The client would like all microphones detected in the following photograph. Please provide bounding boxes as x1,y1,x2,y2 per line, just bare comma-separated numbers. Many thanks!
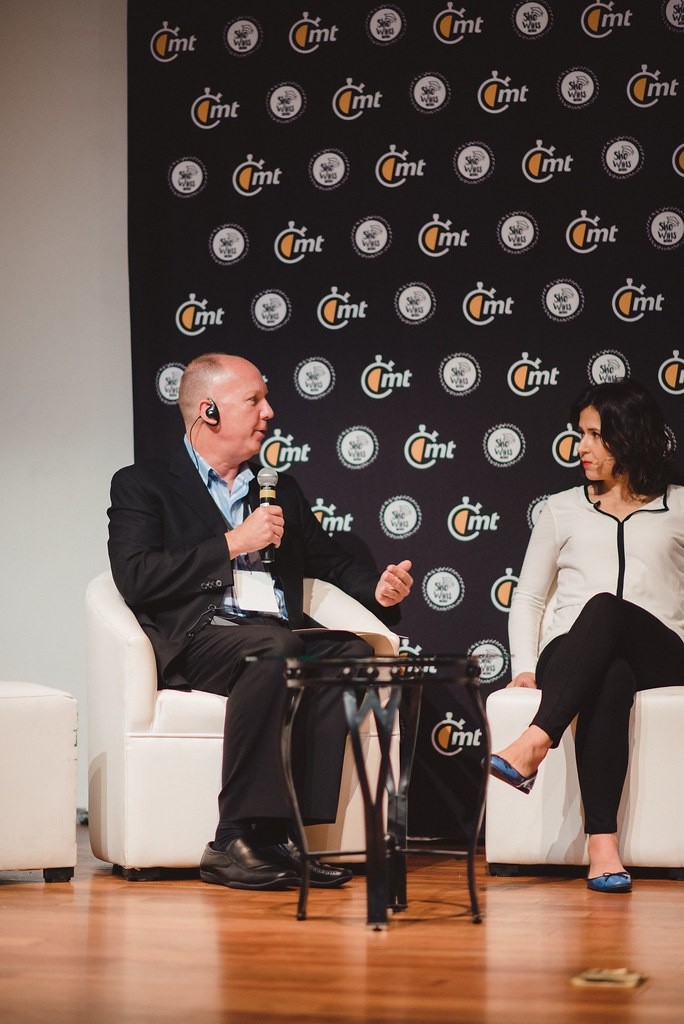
593,458,614,466
256,467,279,563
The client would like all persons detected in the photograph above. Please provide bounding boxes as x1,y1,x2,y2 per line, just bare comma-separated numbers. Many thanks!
107,354,413,886
481,381,684,892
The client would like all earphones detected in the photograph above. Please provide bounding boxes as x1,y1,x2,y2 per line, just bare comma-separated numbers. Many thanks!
206,400,219,422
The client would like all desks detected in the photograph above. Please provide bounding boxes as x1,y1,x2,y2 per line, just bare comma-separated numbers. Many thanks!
284,654,495,932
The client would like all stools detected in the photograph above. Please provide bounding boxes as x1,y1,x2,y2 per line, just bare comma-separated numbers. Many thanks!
485,685,684,880
0,681,79,883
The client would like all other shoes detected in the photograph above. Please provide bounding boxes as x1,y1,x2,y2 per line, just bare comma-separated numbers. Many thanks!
588,863,632,891
481,754,538,793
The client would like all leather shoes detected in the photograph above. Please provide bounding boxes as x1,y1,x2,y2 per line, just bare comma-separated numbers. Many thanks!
200,835,300,890
249,837,350,887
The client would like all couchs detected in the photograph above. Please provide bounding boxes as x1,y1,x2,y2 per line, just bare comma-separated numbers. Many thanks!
89,566,400,882
